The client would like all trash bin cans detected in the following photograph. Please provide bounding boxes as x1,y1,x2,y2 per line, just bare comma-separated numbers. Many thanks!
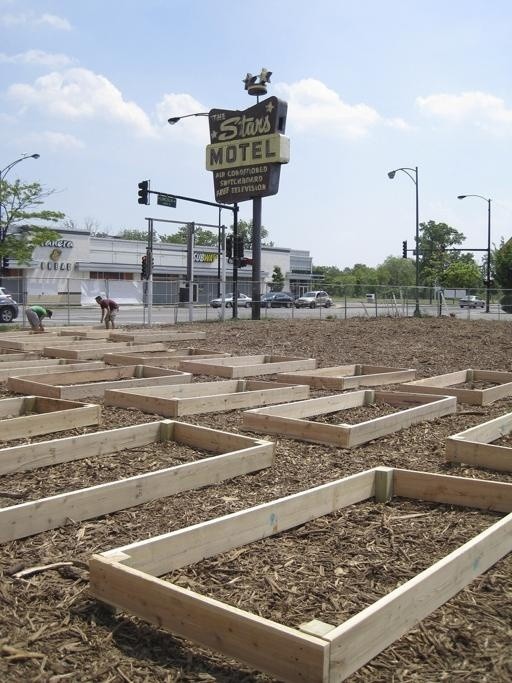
366,294,375,304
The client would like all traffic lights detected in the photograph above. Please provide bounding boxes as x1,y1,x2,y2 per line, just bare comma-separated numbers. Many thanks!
137,178,151,205
402,240,407,258
141,257,147,273
237,236,245,256
151,257,155,268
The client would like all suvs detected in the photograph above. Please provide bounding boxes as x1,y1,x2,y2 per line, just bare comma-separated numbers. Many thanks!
295,289,332,308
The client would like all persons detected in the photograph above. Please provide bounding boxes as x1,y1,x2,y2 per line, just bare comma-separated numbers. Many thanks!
94,296,119,329
25,305,53,330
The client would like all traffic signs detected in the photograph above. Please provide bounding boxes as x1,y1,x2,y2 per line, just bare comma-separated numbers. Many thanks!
156,194,178,208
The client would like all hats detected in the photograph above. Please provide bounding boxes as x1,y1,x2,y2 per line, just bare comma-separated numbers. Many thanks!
47,310,53,319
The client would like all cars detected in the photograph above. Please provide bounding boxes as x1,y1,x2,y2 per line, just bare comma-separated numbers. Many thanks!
458,294,485,308
210,291,253,307
261,291,294,307
0,286,18,323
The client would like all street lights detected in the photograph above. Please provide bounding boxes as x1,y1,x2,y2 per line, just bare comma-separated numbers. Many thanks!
0,151,40,190
387,164,421,317
168,109,240,320
457,193,491,312
